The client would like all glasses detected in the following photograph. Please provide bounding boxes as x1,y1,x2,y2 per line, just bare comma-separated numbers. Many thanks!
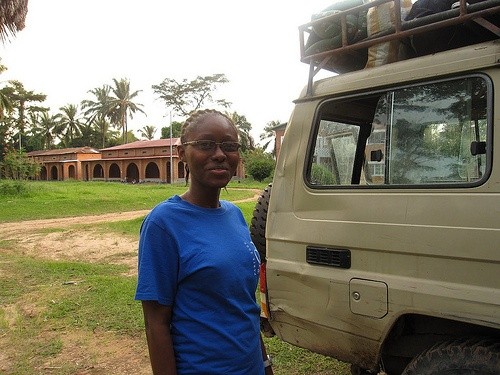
182,140,242,152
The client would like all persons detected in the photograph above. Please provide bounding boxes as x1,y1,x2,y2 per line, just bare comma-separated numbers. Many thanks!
134,108,275,375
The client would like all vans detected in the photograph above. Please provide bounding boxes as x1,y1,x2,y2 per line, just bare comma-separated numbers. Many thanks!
246,0,499,375
